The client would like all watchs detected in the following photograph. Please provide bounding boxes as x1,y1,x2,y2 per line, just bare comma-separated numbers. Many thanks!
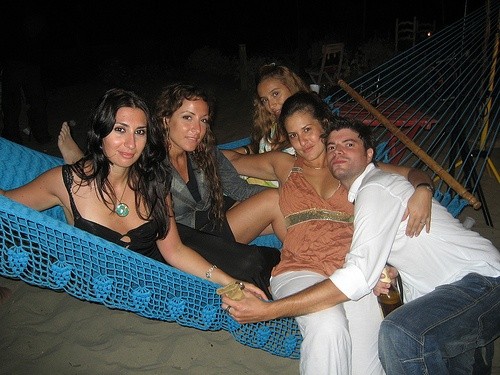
415,182,434,198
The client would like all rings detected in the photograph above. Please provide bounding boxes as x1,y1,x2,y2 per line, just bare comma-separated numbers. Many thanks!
225,305,231,312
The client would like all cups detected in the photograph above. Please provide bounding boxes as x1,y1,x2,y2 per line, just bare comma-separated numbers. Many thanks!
310,84,320,95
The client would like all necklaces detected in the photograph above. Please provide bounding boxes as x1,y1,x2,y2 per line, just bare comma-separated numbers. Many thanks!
303,162,328,169
108,184,129,218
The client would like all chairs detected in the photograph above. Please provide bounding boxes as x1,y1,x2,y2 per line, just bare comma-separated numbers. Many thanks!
395,17,416,51
308,43,344,84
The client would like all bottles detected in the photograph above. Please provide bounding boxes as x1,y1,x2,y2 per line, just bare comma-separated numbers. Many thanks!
377,265,401,318
371,73,380,106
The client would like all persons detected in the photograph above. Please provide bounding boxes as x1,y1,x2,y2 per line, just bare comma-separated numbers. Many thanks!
0,88,281,320
151,82,286,243
222,92,435,375
59,63,309,188
222,116,500,375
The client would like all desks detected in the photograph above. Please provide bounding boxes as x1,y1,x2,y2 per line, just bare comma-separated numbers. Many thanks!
334,97,437,166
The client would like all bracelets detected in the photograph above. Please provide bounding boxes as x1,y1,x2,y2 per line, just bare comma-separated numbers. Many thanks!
235,280,245,290
206,264,217,279
